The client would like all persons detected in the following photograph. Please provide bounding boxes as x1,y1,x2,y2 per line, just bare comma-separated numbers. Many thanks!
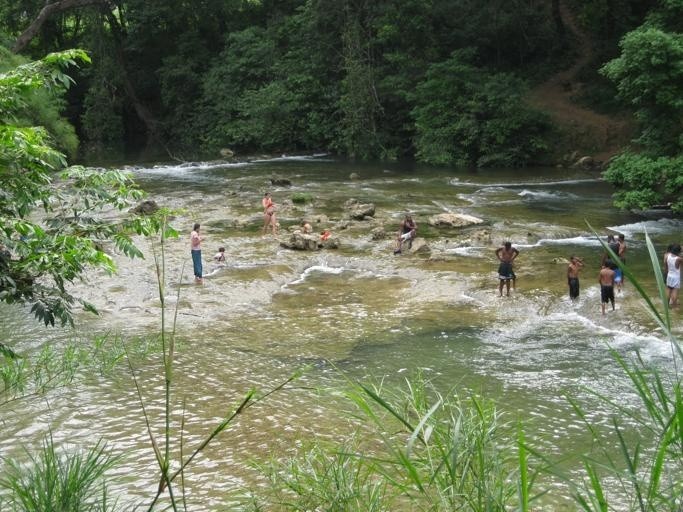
394,215,417,253
496,234,683,315
262,193,276,236
214,248,226,260
191,224,204,280
301,222,313,233
